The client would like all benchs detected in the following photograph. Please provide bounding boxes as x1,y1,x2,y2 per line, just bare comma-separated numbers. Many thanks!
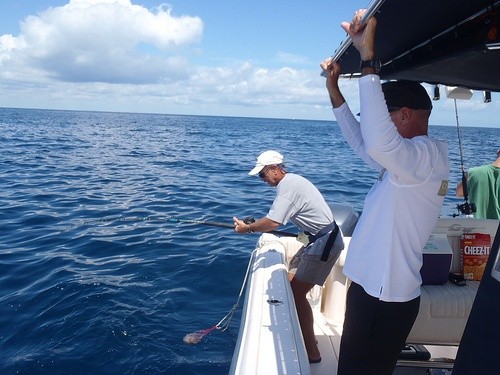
405,236,481,345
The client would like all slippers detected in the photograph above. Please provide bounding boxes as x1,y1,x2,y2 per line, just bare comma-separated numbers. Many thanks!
308,357,321,363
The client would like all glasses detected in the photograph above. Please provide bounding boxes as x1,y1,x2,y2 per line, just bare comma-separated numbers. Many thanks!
259,168,269,178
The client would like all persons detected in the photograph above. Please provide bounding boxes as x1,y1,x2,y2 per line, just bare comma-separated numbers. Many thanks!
233,150,344,364
456,150,500,220
320,8,449,375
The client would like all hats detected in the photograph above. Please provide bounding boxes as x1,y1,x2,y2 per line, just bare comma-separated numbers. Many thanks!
357,80,432,116
248,151,283,175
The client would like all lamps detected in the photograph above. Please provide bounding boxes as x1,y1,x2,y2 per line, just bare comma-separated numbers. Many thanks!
433,84,440,100
482,90,491,103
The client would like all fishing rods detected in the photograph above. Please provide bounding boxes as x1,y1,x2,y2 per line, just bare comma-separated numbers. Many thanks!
454,98,476,215
71,215,313,246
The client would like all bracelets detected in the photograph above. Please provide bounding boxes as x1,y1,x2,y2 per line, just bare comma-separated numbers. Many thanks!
248,225,254,234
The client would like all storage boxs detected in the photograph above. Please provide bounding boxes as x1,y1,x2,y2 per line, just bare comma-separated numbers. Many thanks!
420,233,453,285
460,233,491,280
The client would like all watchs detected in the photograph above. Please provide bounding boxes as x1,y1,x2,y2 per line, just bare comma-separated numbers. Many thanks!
360,56,381,72
